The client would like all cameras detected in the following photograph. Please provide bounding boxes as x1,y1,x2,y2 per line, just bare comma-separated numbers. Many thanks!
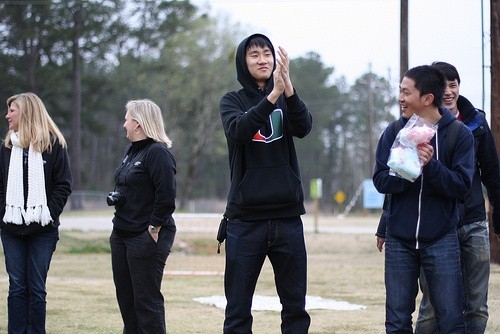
107,191,124,206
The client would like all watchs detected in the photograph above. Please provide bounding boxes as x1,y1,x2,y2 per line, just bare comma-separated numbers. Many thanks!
150,225,159,233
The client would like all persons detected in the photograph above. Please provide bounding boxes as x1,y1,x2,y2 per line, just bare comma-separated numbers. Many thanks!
217,33,313,334
373,62,500,334
0,93,73,334
107,99,177,334
